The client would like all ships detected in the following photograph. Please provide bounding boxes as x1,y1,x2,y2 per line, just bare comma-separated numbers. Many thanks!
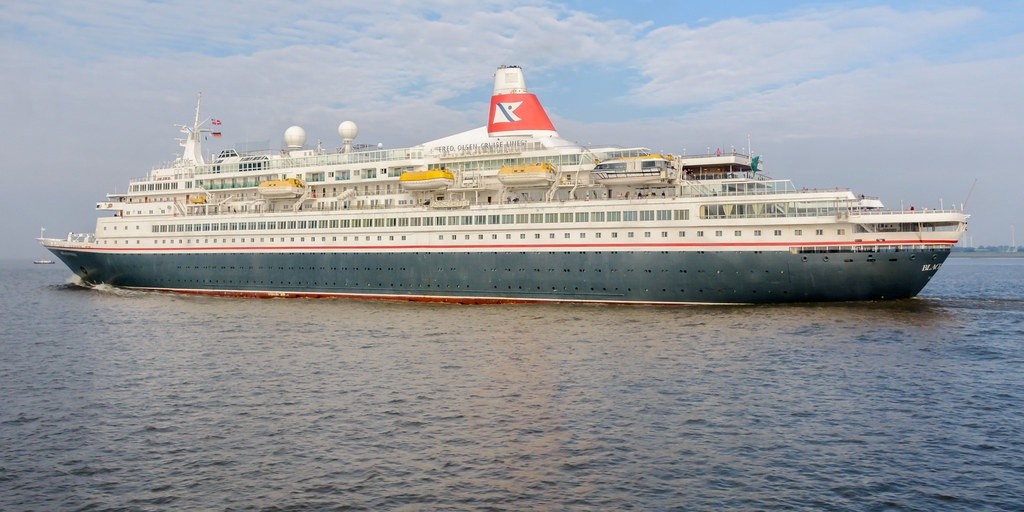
36,65,975,304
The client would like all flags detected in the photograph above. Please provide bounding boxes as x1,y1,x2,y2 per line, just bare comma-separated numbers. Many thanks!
211,119,222,138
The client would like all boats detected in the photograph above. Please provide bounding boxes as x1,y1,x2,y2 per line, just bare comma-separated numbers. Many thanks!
34,259,54,264
398,166,453,193
256,178,307,199
498,163,557,187
591,152,672,185
190,195,207,204
